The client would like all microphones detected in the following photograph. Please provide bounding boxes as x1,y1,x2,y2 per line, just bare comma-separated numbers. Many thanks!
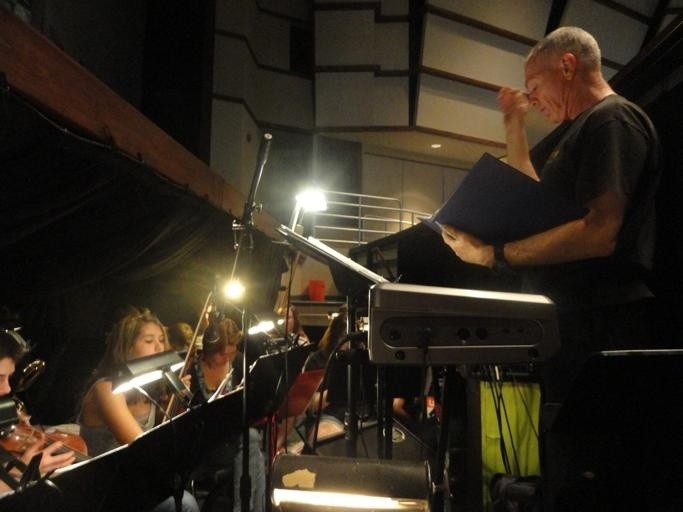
250,130,272,202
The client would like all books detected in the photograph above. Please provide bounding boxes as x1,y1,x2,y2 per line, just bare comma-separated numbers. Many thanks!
416,153,587,249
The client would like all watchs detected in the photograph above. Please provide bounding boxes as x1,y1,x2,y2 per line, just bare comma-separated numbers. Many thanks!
488,241,512,277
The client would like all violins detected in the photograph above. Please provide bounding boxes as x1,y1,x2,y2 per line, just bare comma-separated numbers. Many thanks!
0,419,95,467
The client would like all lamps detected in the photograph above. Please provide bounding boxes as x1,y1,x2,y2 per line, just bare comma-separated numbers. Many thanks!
246,312,286,338
110,349,201,420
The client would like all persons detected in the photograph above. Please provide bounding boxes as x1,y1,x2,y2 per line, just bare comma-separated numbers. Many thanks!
1,330,76,493
431,24,666,512
77,297,445,511
